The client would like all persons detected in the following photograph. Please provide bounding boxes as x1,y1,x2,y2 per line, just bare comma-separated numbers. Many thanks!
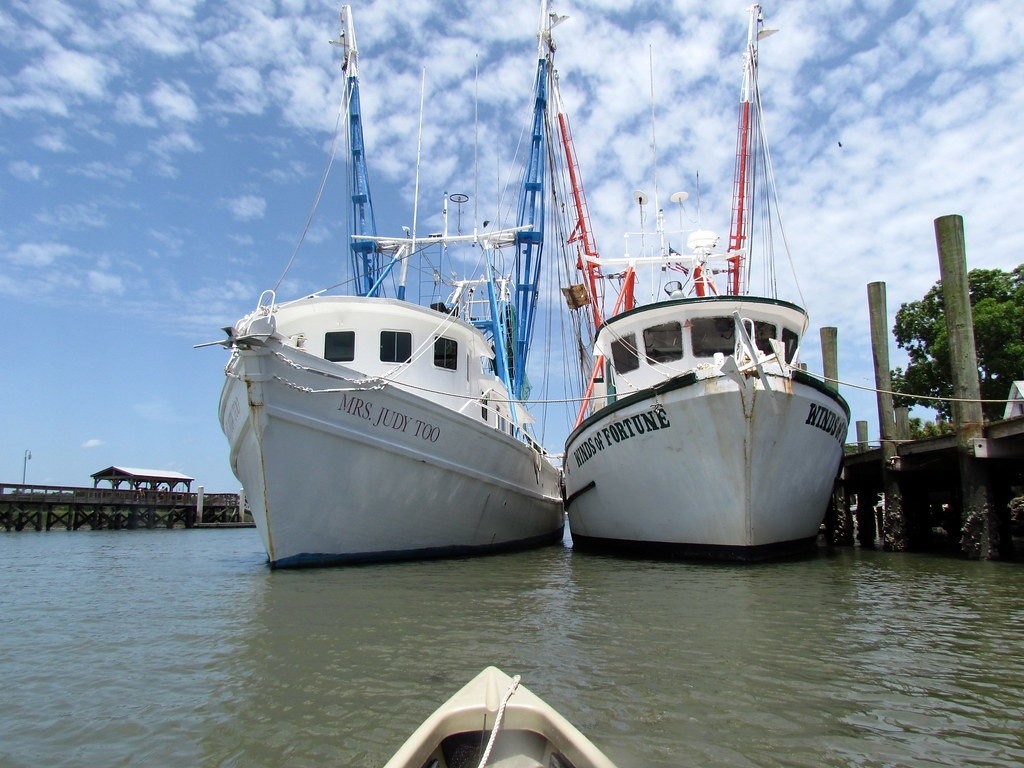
135,485,167,502
935,410,942,425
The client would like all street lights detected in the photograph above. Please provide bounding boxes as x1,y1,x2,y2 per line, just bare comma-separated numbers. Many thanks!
23,449,32,494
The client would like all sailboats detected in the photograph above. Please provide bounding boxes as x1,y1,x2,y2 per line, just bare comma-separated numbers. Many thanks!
553,1,854,567
189,0,568,572
